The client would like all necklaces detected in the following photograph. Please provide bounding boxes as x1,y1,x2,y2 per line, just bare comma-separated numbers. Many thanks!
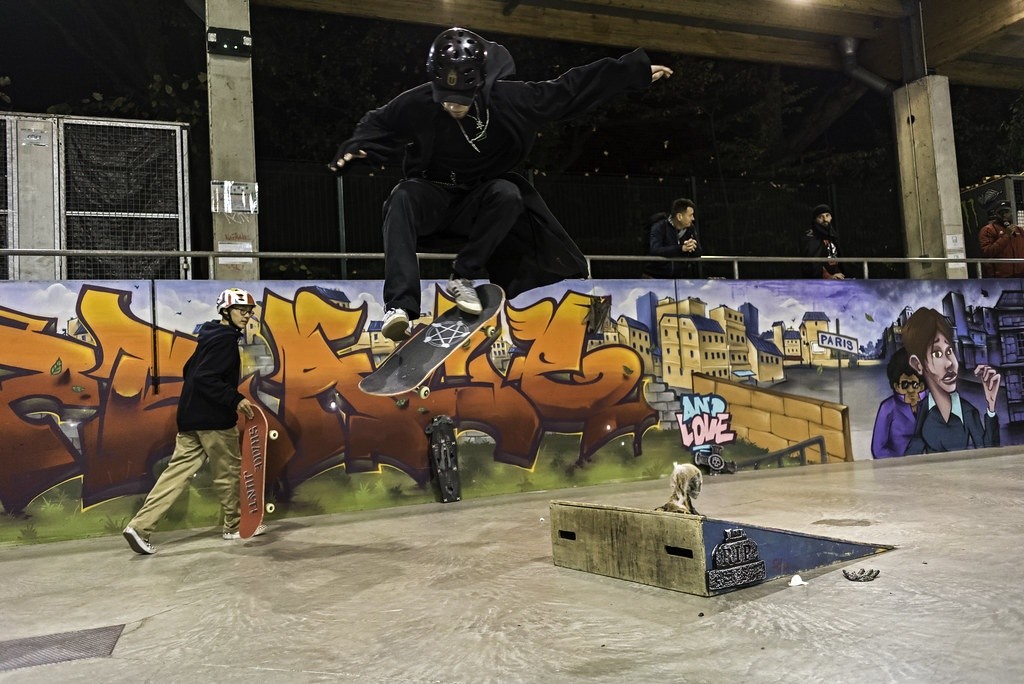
457,100,490,153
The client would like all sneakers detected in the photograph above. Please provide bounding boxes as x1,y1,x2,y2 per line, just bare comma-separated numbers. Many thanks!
381,308,411,342
446,278,482,315
122,527,157,554
222,524,268,539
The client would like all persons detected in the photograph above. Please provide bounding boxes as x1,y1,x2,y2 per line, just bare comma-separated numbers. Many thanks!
978,201,1024,278
638,198,702,279
325,28,671,341
124,288,267,555
799,205,862,280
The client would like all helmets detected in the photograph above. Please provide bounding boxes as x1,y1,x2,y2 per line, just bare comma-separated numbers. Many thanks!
216,288,255,314
427,27,486,90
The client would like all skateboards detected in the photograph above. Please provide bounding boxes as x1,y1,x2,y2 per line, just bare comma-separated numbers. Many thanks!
358,283,506,399
423,413,462,504
238,404,278,541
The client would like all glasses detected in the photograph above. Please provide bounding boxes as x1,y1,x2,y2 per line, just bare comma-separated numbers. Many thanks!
230,306,255,318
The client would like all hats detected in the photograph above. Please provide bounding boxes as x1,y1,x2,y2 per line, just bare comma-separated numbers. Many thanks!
813,205,831,220
994,200,1011,213
432,89,476,106
